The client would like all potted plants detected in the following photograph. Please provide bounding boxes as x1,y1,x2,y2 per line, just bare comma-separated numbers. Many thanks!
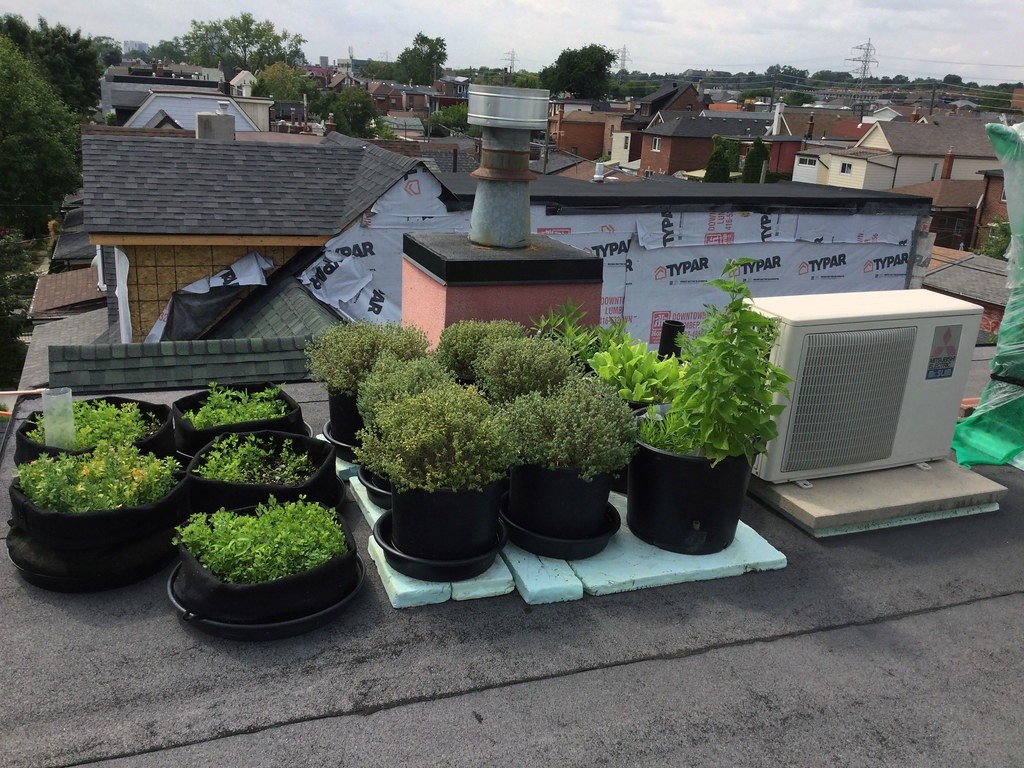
5,258,797,641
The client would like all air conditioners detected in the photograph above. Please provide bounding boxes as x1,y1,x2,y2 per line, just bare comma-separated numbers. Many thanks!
730,288,984,489
